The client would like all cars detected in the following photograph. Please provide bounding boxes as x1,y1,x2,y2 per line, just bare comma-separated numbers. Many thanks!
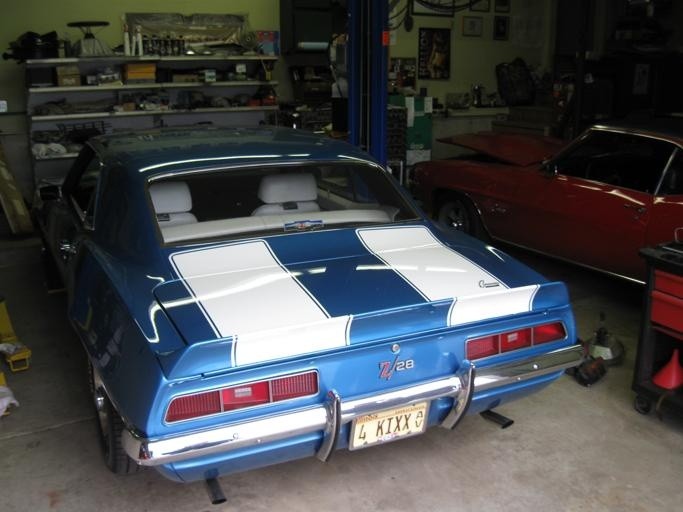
410,121,683,288
36,124,584,485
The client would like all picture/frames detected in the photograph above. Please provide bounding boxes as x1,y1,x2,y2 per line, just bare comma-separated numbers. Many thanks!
492,15,510,41
462,15,483,37
417,27,451,81
411,0,455,18
469,0,510,13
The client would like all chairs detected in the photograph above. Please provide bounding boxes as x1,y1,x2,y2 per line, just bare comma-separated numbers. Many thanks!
148,182,197,229
250,174,321,217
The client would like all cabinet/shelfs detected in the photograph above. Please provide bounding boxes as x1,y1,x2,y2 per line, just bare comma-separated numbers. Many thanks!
24,55,282,207
631,239,682,413
